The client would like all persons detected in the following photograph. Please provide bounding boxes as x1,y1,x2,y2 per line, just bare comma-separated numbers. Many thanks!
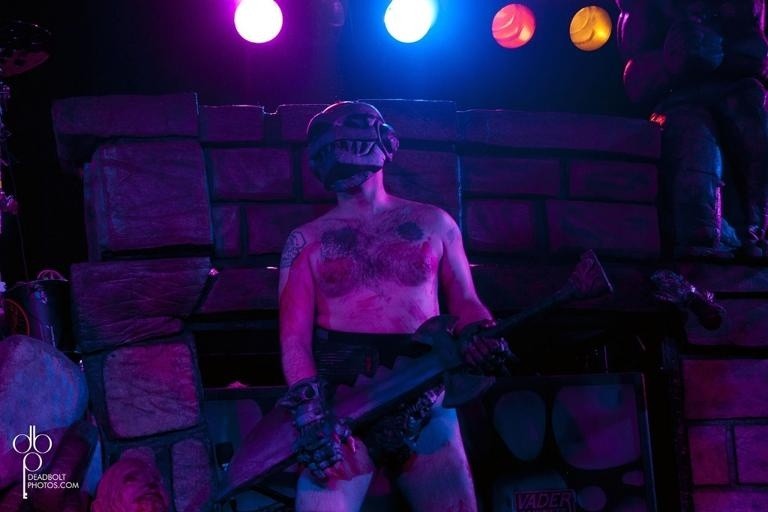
278,103,502,511
91,455,170,512
